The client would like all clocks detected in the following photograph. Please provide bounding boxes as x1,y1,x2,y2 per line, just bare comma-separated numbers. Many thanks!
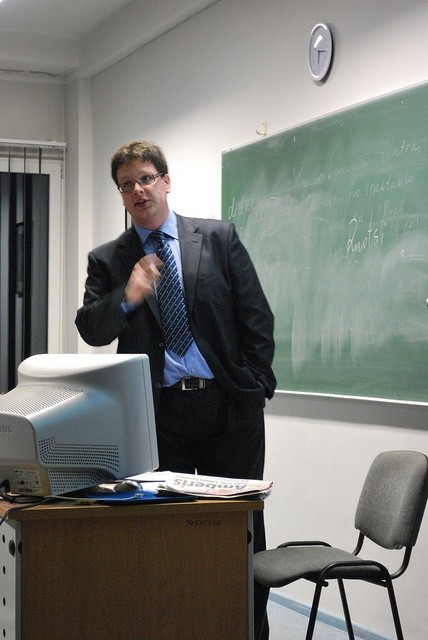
307,22,332,82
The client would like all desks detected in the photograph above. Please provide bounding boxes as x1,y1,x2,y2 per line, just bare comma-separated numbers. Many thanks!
0,496,265,639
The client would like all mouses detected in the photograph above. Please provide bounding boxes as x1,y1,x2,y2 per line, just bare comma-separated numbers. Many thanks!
114,478,143,494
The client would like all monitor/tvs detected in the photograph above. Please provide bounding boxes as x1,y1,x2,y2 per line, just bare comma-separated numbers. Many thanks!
0,353,159,497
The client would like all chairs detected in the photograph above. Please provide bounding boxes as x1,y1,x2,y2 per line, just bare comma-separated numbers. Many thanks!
252,450,428,640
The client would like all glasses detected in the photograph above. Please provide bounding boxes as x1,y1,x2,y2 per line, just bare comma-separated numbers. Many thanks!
118,171,165,194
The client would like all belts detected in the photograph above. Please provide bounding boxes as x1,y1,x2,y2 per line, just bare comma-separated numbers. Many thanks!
177,376,216,391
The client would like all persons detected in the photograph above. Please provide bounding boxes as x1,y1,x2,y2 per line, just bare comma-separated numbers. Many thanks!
75,142,277,635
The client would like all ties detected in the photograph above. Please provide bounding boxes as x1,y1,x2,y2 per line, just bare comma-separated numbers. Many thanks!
150,231,193,358
